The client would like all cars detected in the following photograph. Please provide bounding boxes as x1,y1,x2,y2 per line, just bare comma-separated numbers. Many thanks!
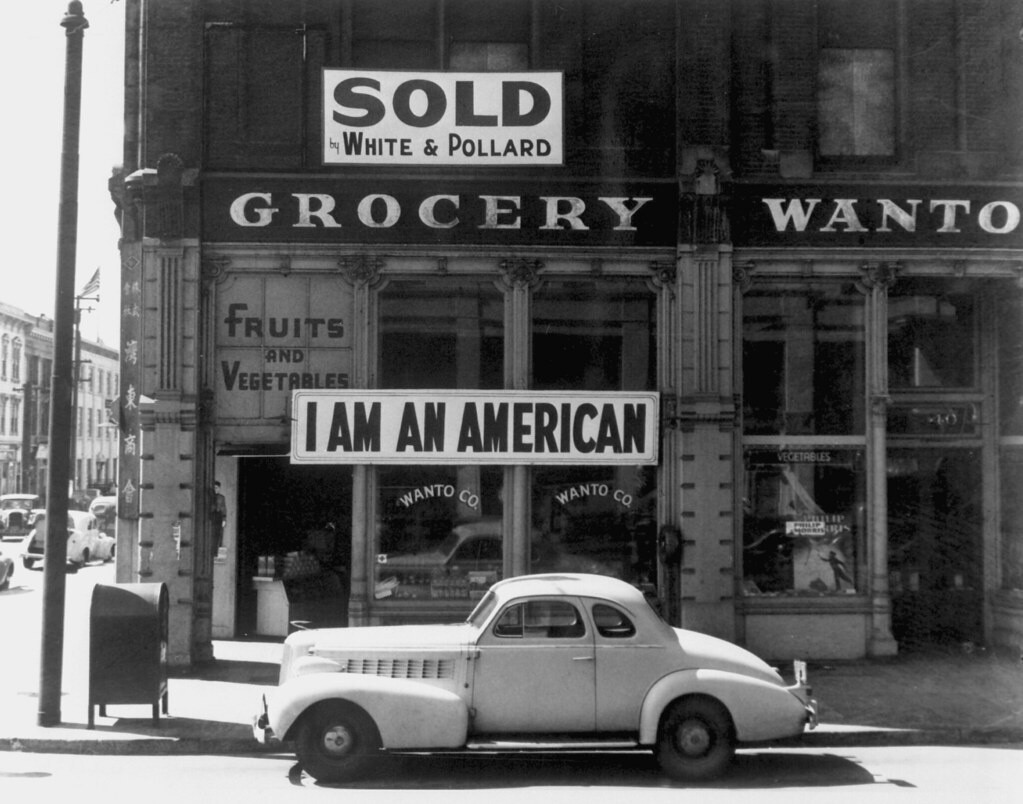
0,492,46,540
19,509,113,569
378,518,625,583
88,496,121,537
249,570,820,782
1,552,15,591
